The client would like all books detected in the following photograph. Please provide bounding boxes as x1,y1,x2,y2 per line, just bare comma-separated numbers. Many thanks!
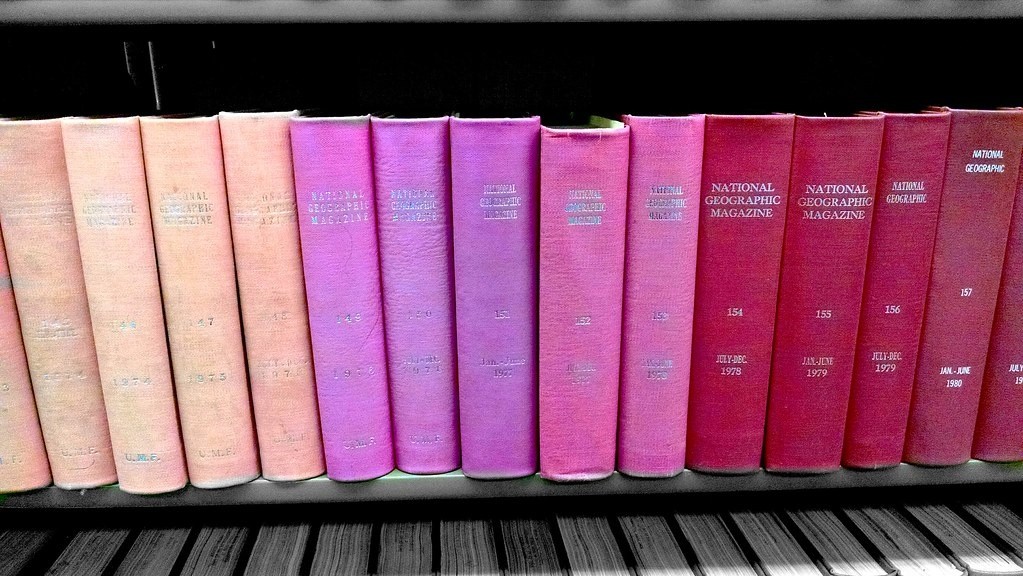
61,115,187,494
1,503,1023,576
137,103,1023,494
0,115,116,490
0,235,52,493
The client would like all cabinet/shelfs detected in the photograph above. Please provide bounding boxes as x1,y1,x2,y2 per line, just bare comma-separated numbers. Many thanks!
0,0,1023,510
